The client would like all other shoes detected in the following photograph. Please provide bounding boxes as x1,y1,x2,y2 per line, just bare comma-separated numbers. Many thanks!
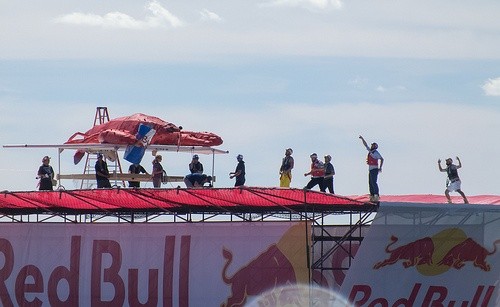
448,201,452,203
465,200,468,204
370,194,380,201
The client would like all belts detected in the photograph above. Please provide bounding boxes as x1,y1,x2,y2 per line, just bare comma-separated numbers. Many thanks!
452,179,459,183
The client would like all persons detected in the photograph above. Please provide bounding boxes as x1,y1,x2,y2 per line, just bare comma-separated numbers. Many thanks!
189,154,203,186
229,154,246,187
324,154,335,194
185,173,212,188
127,163,150,187
438,156,469,204
279,148,294,188
38,156,54,190
359,136,384,199
95,153,112,188
302,152,326,192
152,155,166,188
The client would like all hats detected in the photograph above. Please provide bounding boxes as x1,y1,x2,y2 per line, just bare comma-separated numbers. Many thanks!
446,158,453,162
310,153,317,158
193,154,198,159
207,176,212,183
237,154,243,159
96,154,102,159
42,156,51,161
324,155,332,160
286,148,293,154
371,143,378,149
